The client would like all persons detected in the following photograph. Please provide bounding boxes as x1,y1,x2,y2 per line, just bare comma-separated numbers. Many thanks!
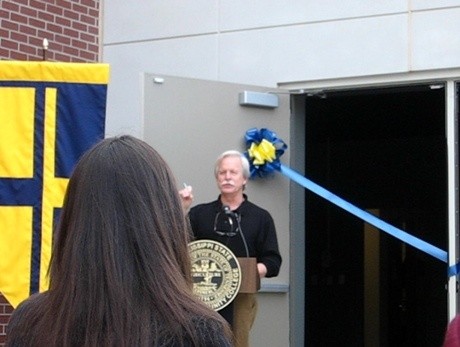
4,133,234,347
176,148,283,347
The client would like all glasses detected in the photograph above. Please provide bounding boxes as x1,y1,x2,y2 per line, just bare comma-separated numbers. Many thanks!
214,211,241,237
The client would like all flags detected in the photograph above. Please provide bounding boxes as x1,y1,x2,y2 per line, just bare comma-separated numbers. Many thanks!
0,61,111,311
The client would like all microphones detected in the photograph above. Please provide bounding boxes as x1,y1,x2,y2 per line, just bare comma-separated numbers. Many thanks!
222,205,234,230
